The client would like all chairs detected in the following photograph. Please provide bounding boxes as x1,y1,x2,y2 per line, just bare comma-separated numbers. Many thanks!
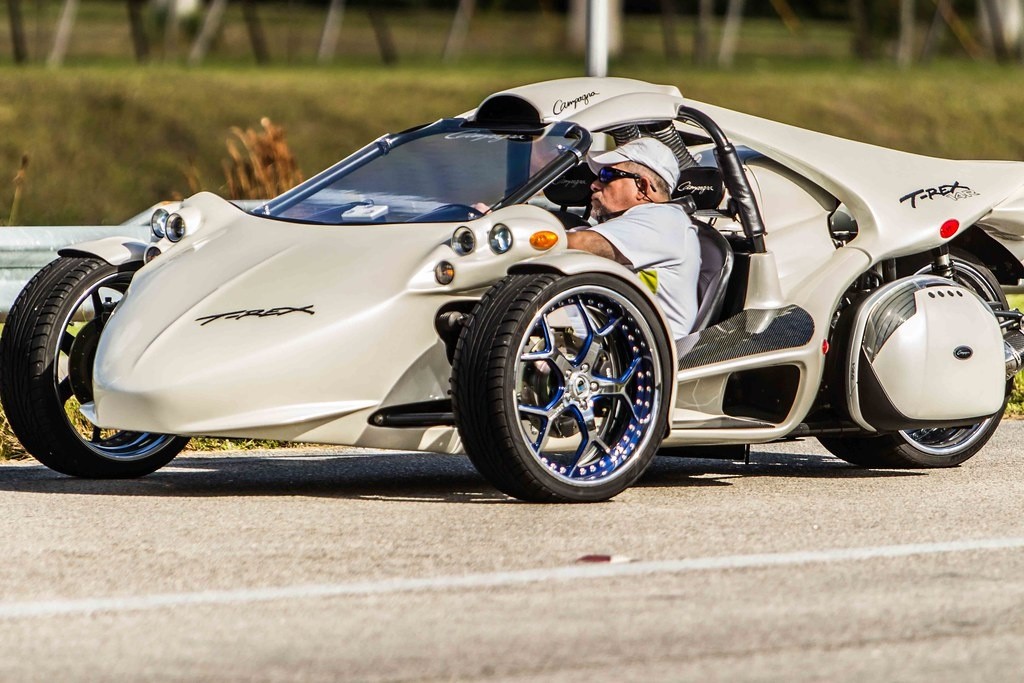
543,162,599,230
671,166,734,334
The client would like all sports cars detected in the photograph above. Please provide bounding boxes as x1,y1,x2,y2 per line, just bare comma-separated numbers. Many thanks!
0,74,1024,502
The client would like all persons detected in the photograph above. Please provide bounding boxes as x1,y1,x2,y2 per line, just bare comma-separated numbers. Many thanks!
469,136,701,340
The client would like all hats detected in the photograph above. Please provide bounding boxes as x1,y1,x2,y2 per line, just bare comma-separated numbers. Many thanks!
587,138,681,195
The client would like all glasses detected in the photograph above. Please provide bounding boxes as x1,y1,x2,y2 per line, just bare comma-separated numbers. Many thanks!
598,167,656,192
